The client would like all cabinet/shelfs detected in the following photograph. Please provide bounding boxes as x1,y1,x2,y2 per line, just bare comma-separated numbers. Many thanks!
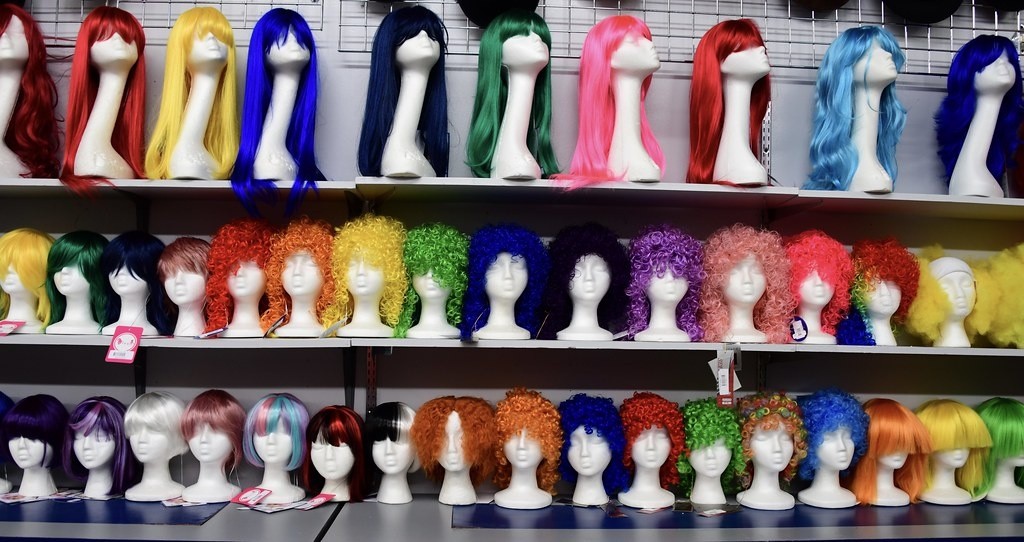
0,177,1024,542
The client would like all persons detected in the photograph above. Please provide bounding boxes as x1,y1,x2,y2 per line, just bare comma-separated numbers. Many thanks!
0,6,1024,508
